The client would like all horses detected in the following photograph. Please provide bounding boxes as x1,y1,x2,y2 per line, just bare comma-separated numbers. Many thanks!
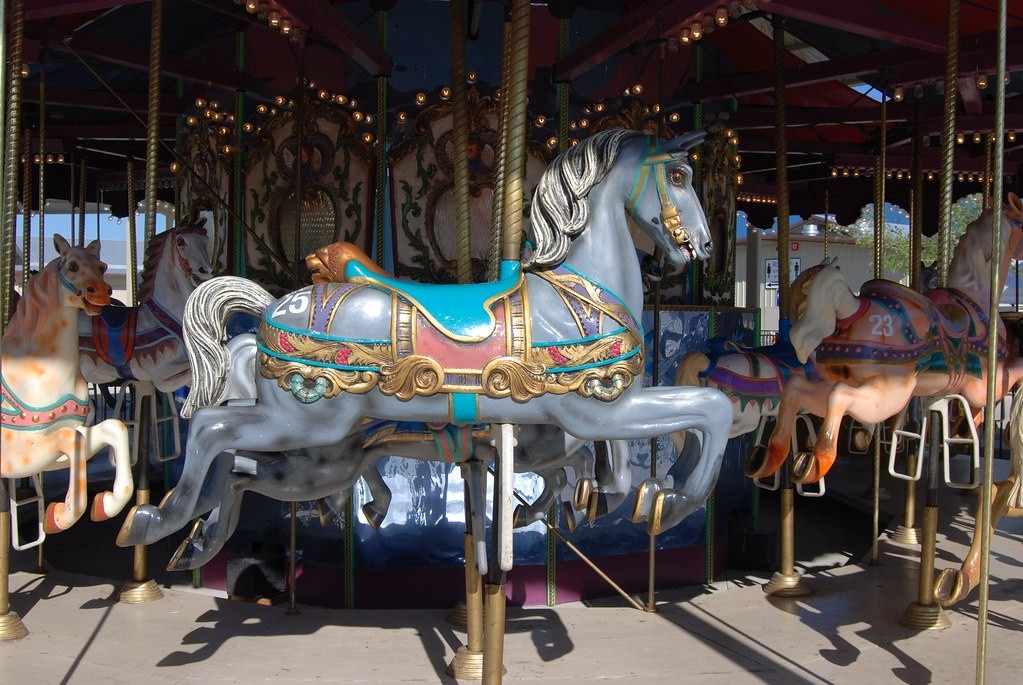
667,191,1023,484
115,128,734,572
0,214,214,534
